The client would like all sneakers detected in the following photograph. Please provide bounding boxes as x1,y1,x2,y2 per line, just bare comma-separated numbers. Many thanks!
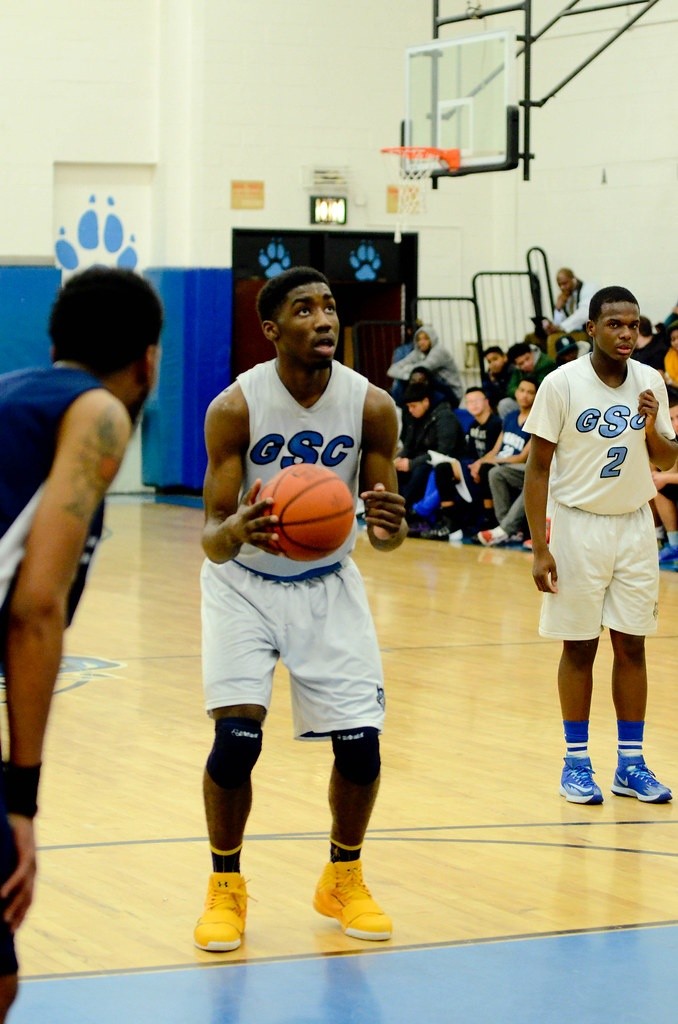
312,859,392,940
610,761,672,802
559,757,601,804
478,526,508,546
524,519,550,549
193,872,257,951
505,532,524,542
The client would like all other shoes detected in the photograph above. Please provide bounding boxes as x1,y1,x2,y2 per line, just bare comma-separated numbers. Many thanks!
658,542,678,563
449,529,463,540
408,524,431,538
421,527,450,540
471,534,480,543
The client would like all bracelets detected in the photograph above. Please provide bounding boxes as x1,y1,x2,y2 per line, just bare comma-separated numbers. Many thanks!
2,760,42,819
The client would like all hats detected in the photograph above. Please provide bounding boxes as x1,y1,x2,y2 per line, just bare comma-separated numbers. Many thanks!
557,335,578,356
396,383,431,405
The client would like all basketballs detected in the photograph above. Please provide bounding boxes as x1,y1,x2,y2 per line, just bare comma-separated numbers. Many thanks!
259,463,358,564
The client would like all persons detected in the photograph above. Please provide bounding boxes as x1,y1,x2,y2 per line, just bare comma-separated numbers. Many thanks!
522,287,678,802
0,270,161,1024
193,266,408,952
343,267,678,571
